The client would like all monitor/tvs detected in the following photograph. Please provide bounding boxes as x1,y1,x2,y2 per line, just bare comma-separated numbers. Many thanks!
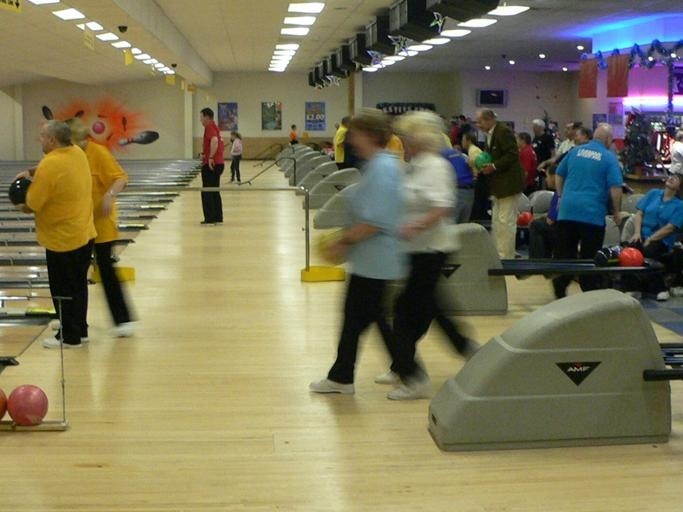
476,87,509,107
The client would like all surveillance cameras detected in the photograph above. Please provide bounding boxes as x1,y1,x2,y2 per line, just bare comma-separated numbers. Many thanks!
118,25,127,33
172,63,177,67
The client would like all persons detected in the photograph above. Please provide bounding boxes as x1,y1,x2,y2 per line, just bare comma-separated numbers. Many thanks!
224,125,243,185
200,107,225,225
11,120,98,350
624,172,683,302
529,165,558,258
289,124,299,145
661,125,683,199
325,112,485,224
504,117,620,197
48,118,141,338
308,108,433,402
553,125,625,299
475,108,525,260
373,112,482,386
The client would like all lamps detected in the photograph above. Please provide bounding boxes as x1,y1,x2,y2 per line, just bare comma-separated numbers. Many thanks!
308,0,533,88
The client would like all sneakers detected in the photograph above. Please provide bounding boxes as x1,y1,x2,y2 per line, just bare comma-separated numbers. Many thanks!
623,292,641,300
228,181,241,184
309,378,355,394
43,321,89,348
375,370,402,384
656,291,670,300
113,321,135,337
670,286,683,296
386,383,433,400
200,220,223,226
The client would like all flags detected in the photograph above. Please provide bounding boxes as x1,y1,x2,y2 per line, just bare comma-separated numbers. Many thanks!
607,55,629,97
578,59,598,98
